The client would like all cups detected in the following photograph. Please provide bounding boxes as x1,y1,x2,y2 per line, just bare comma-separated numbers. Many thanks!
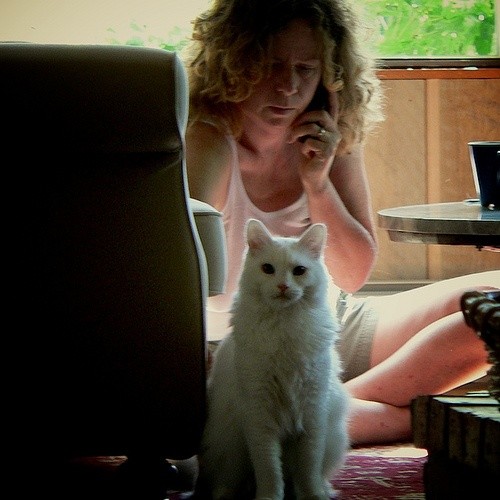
467,141,499,210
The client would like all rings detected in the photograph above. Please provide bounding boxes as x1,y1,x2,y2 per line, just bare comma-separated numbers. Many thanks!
317,128,326,137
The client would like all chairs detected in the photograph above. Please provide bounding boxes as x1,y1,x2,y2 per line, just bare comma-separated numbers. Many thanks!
0,41,227,499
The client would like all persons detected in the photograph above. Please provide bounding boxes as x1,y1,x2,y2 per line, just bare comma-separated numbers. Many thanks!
185,0,499,449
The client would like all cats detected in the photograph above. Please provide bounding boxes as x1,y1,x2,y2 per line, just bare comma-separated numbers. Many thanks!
175,219,352,500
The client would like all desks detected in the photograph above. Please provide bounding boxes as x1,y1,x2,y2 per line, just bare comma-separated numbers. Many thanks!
377,199,500,252
